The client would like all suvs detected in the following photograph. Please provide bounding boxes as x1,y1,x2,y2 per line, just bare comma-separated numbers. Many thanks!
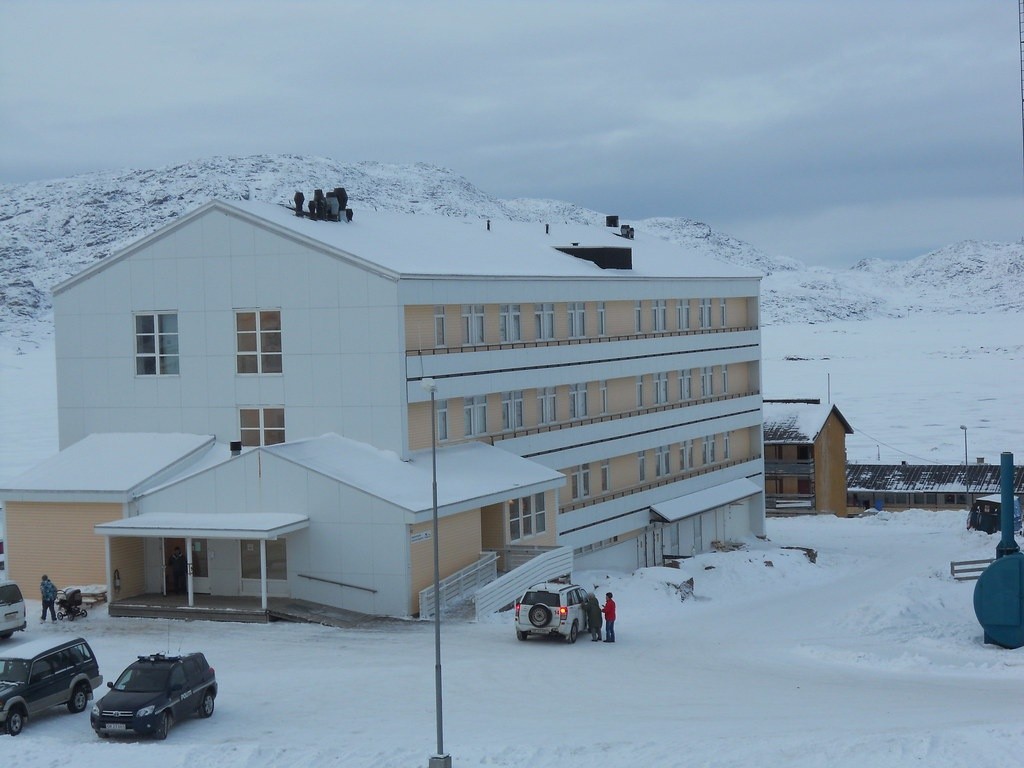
515,582,591,644
0,636,103,736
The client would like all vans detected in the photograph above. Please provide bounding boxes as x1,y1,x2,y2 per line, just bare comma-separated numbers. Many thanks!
0,580,26,640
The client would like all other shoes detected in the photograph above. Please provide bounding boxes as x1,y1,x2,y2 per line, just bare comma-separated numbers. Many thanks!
40,619,46,625
590,637,602,642
603,639,614,643
51,619,58,623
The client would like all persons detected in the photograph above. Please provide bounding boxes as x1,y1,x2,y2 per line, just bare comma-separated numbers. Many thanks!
168,546,187,593
39,575,58,624
583,593,615,642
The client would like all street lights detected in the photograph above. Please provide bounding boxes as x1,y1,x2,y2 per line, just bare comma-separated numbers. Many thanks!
960,424,969,512
419,378,452,768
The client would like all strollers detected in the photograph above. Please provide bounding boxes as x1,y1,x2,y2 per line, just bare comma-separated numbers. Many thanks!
56,587,87,621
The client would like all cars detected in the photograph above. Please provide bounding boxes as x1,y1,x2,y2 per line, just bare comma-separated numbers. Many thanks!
90,651,217,740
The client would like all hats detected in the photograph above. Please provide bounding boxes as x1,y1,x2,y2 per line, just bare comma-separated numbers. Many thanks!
605,592,613,597
